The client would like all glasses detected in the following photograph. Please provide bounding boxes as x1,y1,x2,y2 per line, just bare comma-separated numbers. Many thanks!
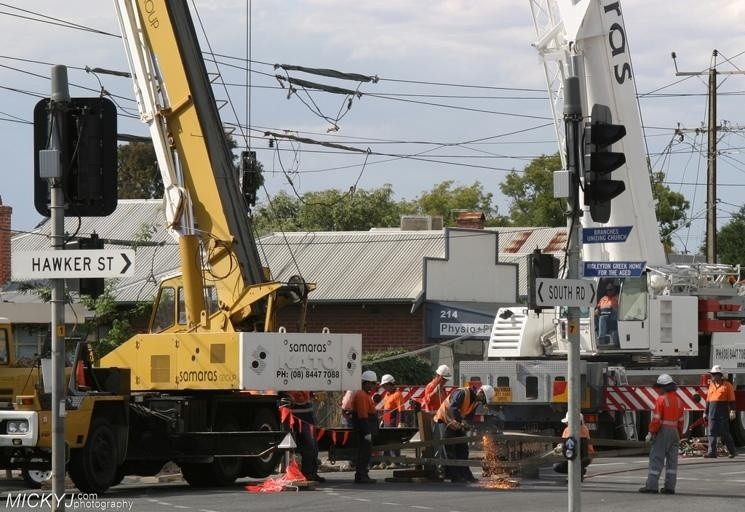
372,382,376,385
443,378,447,380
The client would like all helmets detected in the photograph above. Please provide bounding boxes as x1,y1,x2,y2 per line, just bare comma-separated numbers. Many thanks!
436,364,452,380
361,371,377,382
482,385,495,404
708,365,723,374
561,412,583,423
381,374,395,386
606,283,615,289
657,373,673,385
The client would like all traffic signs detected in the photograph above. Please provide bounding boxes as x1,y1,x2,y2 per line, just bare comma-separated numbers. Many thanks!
533,275,598,309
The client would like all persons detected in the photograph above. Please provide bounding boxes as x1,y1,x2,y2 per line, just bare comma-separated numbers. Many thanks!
342,371,377,484
434,385,496,483
380,374,405,469
703,365,737,458
423,365,453,411
287,391,326,482
552,411,594,482
638,373,684,494
596,284,619,339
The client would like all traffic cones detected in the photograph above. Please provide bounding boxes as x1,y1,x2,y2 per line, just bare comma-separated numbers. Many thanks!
75,358,91,391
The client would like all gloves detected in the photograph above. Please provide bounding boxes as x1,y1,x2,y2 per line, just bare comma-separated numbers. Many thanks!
730,410,736,420
645,433,655,443
703,413,708,421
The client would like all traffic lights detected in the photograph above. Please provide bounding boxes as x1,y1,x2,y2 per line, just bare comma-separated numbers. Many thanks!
583,103,628,223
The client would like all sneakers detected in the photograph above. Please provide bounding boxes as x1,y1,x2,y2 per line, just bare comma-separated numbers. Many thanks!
639,488,659,494
704,453,718,458
727,452,736,458
309,476,325,483
661,489,675,494
386,462,401,469
355,474,376,484
372,463,387,470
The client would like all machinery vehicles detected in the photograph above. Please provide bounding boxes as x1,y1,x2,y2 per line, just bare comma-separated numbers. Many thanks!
372,1,745,457
0,0,440,495
